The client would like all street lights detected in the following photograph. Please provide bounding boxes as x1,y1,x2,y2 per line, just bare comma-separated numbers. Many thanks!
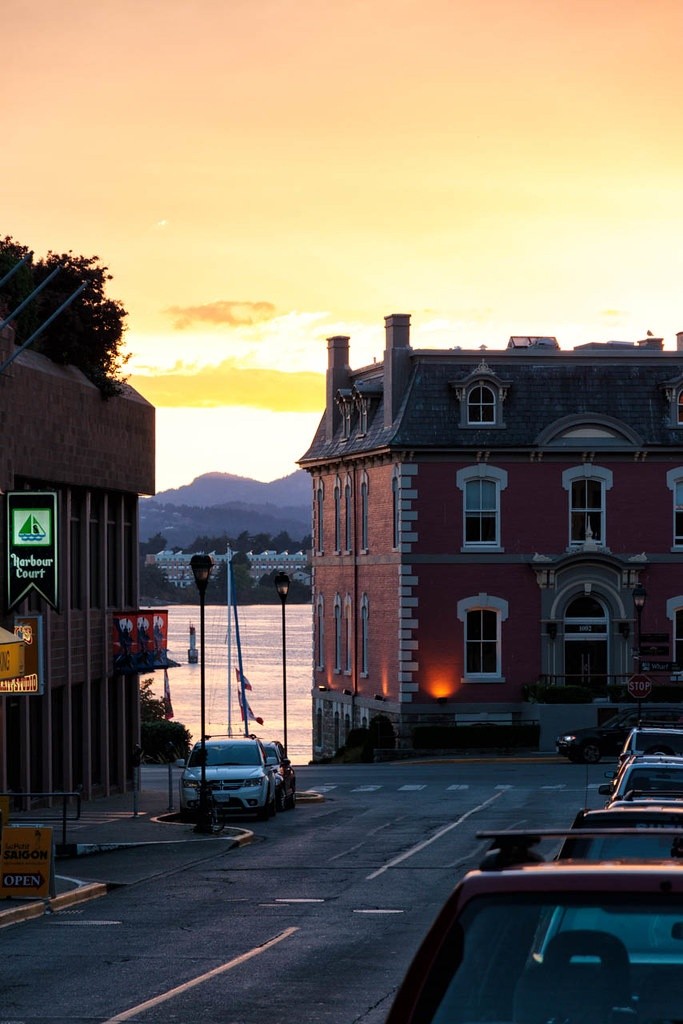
630,583,651,730
188,555,216,834
274,571,292,759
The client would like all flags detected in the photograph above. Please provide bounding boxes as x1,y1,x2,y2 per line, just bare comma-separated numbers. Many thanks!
162,668,174,720
236,667,253,691
237,689,265,725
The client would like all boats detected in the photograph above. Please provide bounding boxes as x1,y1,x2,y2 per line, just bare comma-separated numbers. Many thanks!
188,618,198,664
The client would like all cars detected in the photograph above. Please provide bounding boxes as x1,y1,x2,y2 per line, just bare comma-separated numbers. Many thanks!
385,828,683,1024
553,809,683,868
598,719,683,811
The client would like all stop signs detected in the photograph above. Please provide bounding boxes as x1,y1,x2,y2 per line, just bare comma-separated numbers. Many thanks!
628,674,652,698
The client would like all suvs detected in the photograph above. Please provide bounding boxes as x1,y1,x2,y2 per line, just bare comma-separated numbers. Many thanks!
555,708,683,765
256,737,296,818
176,734,277,822
